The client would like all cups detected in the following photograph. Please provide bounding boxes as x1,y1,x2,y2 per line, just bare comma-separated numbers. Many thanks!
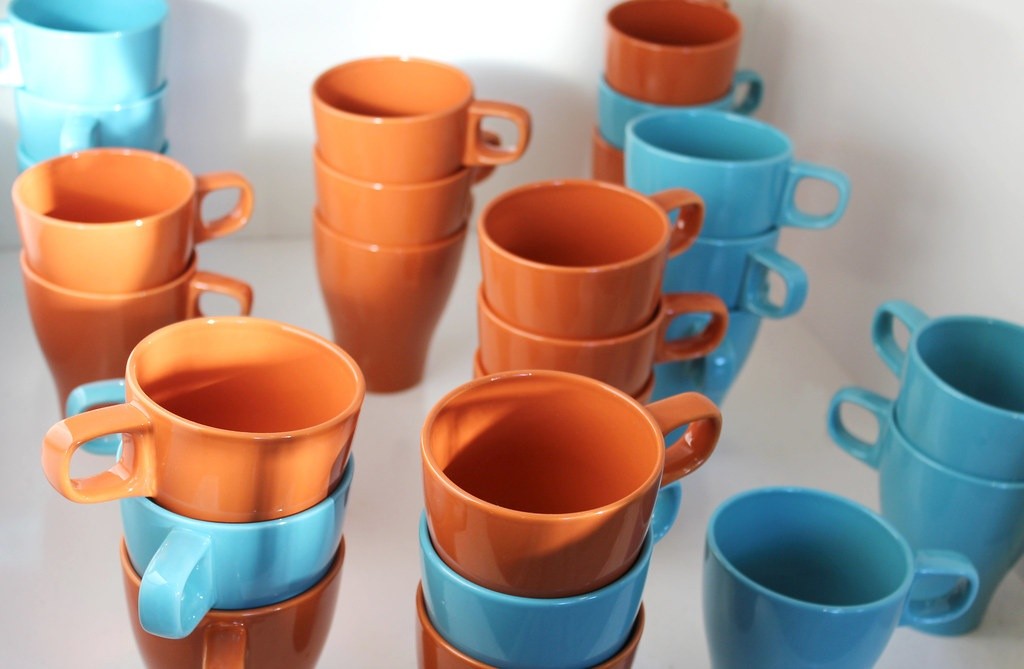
65,378,125,456
474,178,729,407
827,299,1023,637
703,485,980,669
0,0,170,174
12,147,254,419
624,109,851,405
312,56,531,394
592,0,765,187
416,370,722,669
41,315,365,668
